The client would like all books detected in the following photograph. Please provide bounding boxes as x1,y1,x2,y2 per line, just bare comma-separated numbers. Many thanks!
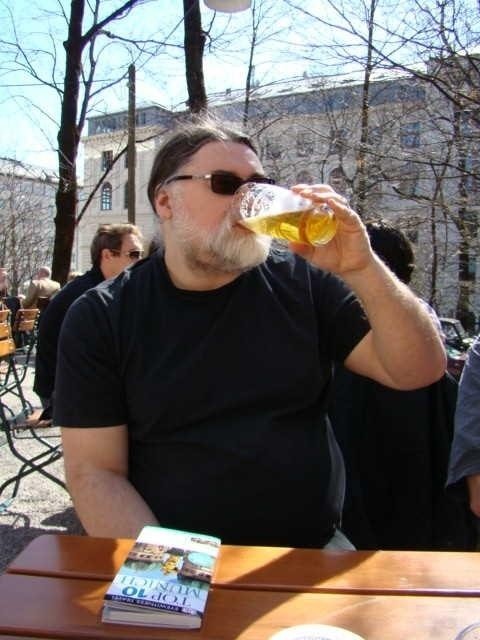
100,524,222,631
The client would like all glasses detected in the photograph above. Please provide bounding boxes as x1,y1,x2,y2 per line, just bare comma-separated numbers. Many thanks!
164,172,278,198
110,248,145,259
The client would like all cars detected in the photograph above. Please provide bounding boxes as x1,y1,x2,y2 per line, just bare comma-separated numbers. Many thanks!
438,318,473,384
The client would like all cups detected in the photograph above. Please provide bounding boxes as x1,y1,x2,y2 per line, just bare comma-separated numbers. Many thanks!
232,181,337,250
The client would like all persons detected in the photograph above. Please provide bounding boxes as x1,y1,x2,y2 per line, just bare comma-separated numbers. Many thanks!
54,120,450,555
0,267,21,329
12,266,60,353
36,221,149,422
325,220,480,553
444,327,480,520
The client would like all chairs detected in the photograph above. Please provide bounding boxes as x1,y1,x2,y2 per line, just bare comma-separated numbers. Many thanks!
0,309,75,499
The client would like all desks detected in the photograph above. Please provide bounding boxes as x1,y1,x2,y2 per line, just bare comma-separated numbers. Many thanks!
0,534,480,640
